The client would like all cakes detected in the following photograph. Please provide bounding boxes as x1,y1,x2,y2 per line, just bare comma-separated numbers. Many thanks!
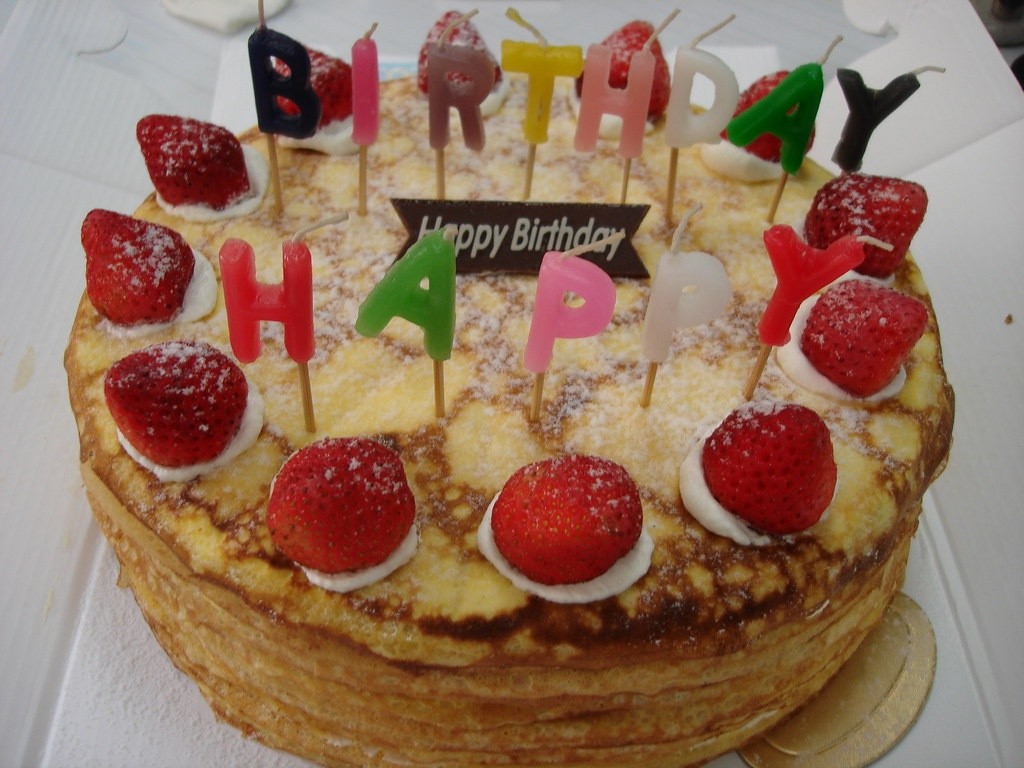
62,0,959,768
